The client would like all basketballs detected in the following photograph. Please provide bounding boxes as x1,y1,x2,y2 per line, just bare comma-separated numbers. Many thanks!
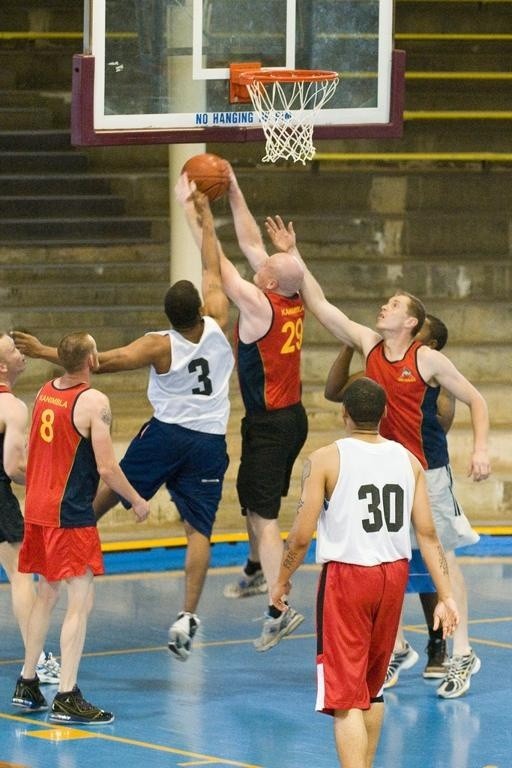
180,152,233,198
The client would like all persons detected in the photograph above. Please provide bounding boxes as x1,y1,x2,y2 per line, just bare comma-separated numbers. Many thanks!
325,313,458,678
377,690,481,768
0,332,64,685
271,375,459,768
19,181,234,662
174,162,308,649
11,332,152,724
263,214,490,702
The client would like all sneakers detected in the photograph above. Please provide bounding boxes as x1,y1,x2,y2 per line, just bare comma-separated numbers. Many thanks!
255,606,304,652
222,569,267,596
382,624,481,698
167,612,200,662
12,650,115,724
